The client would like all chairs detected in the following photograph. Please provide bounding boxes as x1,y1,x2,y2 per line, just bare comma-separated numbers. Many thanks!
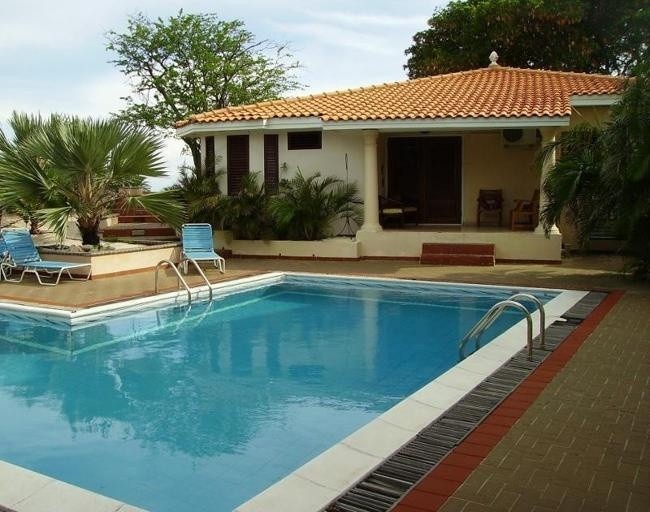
378,195,419,228
476,189,504,227
179,222,227,274
1,226,93,286
509,189,540,231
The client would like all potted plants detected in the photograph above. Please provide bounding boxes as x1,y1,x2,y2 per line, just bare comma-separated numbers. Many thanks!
121,174,146,196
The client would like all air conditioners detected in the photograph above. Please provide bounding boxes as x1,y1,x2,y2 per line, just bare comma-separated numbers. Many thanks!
500,128,538,149
